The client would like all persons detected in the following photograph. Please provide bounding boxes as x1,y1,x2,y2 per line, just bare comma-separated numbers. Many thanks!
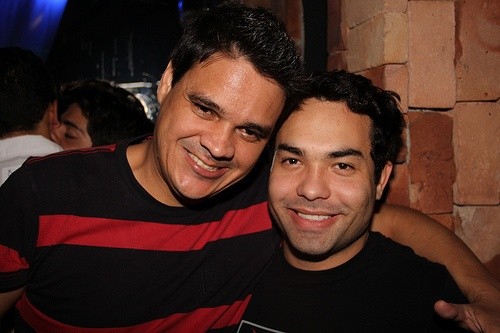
0,0,500,333
238,70,470,333
50,80,150,151
0,46,63,185
